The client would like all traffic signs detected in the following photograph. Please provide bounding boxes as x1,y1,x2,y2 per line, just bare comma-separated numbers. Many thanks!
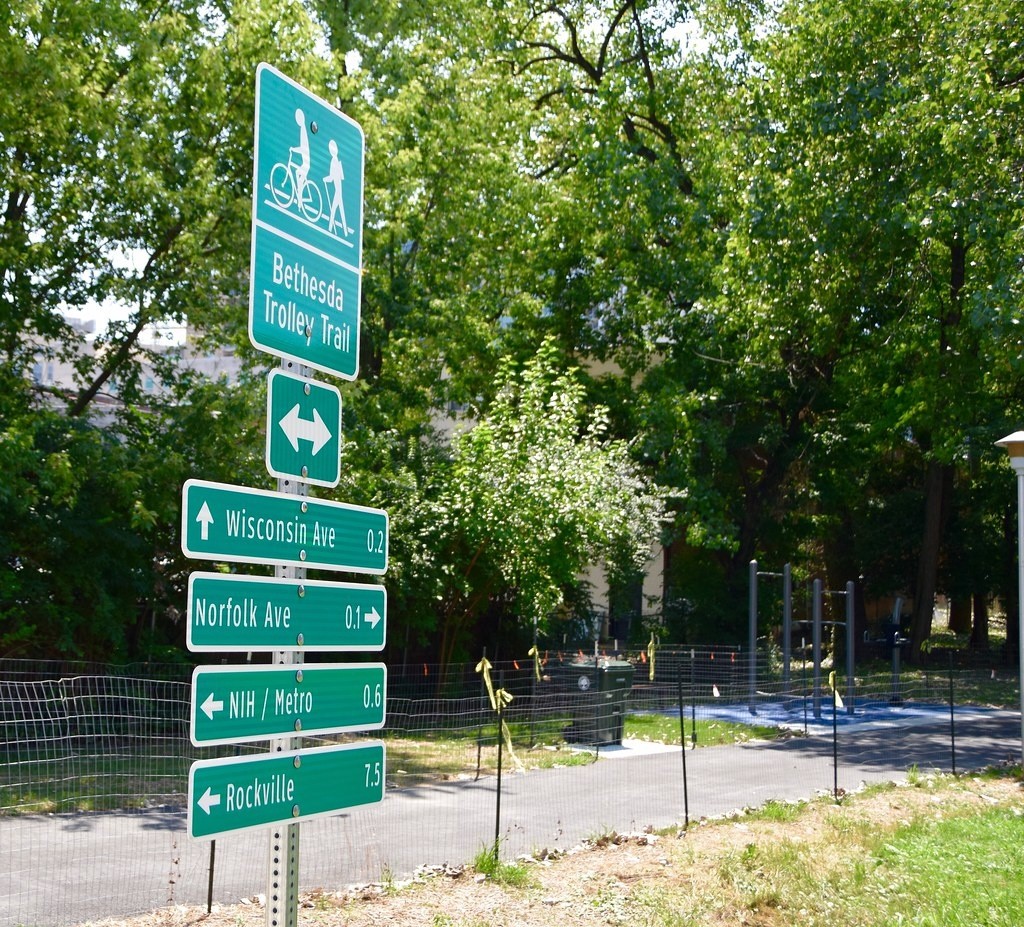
180,476,389,840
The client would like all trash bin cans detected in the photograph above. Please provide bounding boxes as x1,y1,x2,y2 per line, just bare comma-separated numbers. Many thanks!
564,656,637,745
